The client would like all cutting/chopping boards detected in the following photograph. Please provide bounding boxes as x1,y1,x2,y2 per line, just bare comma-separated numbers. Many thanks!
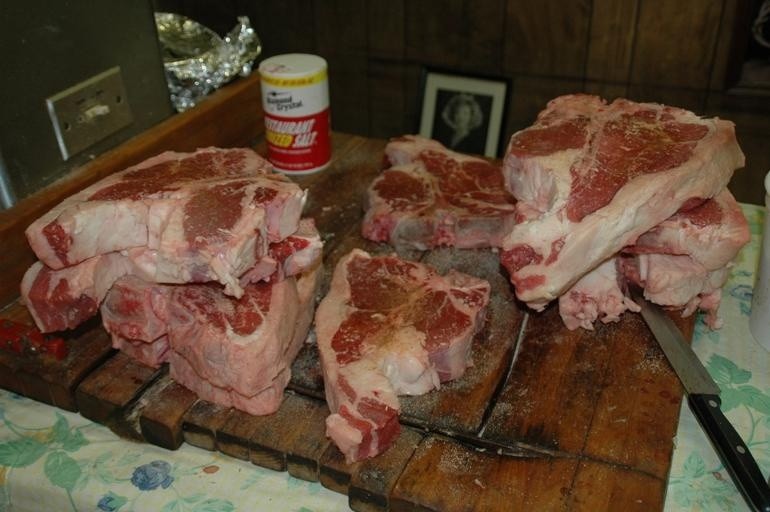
0,77,693,512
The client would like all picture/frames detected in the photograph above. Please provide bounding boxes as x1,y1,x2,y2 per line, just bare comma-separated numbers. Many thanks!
420,73,506,159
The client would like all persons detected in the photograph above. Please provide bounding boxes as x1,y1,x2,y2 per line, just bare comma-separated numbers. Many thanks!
440,93,487,155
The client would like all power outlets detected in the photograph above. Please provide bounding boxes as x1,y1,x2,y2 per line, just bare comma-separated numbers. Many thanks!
46,65,136,161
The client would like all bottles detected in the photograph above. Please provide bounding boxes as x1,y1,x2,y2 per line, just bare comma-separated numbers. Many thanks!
257,53,334,176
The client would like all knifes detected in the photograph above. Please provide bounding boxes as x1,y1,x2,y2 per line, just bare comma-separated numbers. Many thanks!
643,299,770,508
